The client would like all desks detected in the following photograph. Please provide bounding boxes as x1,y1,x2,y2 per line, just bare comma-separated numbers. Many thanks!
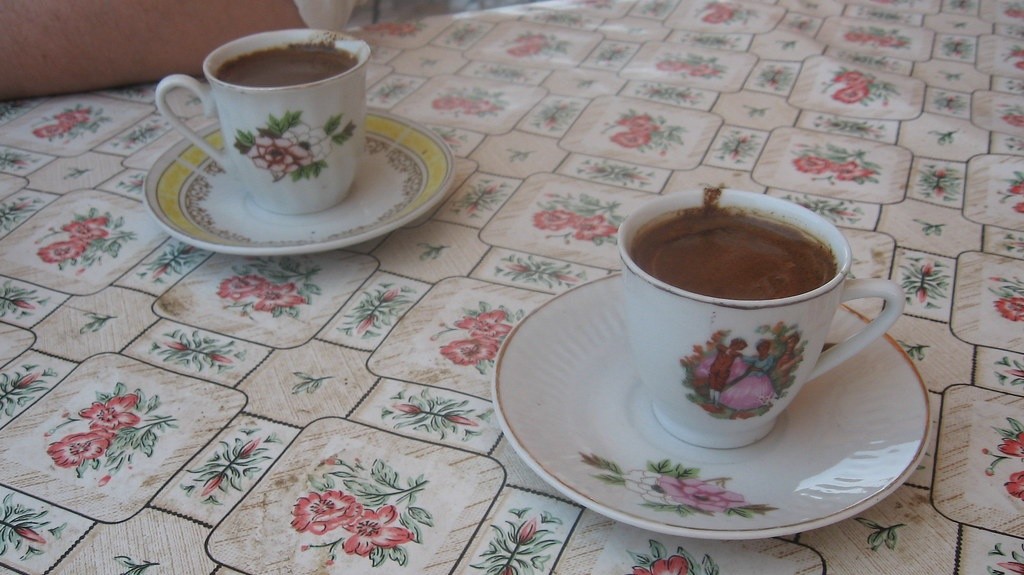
0,0,1024,575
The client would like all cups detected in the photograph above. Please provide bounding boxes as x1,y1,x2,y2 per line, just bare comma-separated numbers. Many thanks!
617,187,905,450
154,27,372,215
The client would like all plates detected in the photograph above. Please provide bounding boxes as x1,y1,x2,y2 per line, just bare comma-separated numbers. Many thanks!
489,273,930,541
144,106,456,254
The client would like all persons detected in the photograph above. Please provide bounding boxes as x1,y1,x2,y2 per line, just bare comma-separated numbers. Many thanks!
0,0,361,99
